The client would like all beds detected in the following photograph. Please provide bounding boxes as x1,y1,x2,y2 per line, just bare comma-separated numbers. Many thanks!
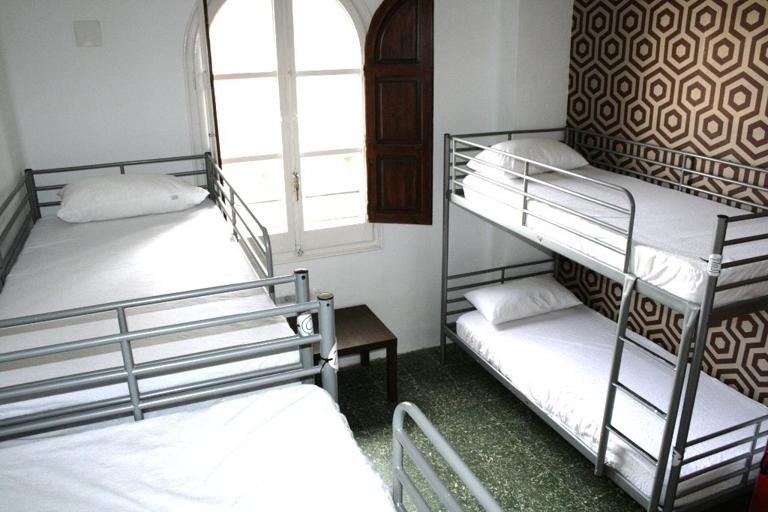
0,292,502,512
440,125,768,512
0,152,315,438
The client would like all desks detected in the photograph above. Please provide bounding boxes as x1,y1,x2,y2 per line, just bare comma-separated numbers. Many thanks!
287,304,398,401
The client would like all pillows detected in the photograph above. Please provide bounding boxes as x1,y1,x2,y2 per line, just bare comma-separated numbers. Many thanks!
465,137,590,179
56,173,210,223
463,273,583,326
